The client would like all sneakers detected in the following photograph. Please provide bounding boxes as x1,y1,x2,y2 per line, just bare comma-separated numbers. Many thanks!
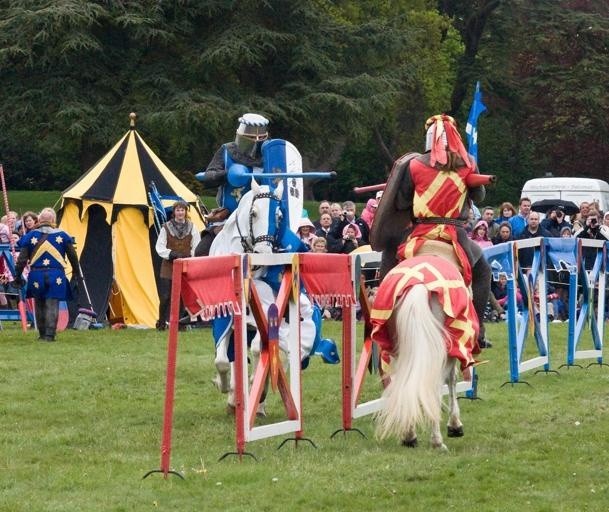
481,309,581,321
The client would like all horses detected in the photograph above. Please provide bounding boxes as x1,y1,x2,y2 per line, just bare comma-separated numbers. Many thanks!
368,254,466,453
194,174,309,418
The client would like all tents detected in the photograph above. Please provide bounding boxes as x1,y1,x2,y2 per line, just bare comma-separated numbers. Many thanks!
53,121,212,330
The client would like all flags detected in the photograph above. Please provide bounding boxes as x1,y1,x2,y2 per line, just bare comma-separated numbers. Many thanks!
464,80,488,165
146,181,167,222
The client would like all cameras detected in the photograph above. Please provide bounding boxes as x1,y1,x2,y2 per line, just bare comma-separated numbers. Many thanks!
556,205,565,218
591,218,597,225
344,234,354,241
341,210,354,217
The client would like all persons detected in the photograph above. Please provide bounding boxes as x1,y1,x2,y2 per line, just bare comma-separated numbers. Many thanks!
298,189,385,322
155,201,203,332
193,113,307,323
374,113,494,353
466,196,609,325
0,206,76,343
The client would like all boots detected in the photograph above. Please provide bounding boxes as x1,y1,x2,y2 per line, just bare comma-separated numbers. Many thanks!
478,326,493,348
39,331,56,342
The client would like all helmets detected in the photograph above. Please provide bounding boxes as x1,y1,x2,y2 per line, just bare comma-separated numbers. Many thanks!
424,113,457,153
234,112,270,160
